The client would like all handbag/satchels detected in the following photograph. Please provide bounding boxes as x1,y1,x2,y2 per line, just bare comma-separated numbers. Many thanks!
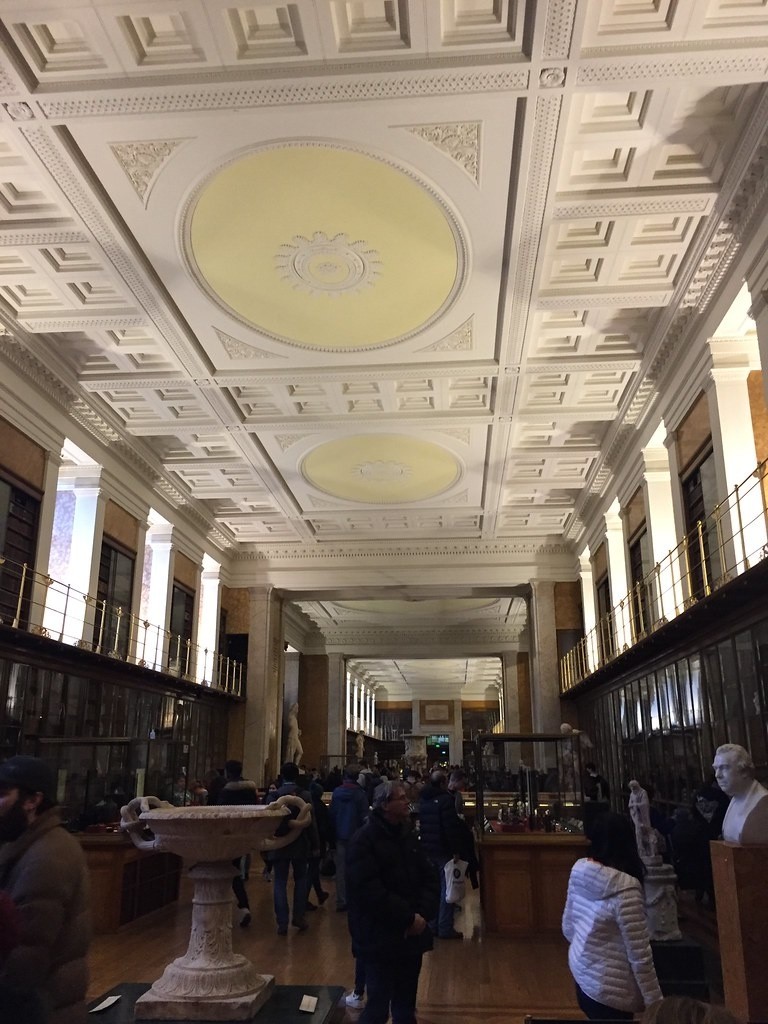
443,858,468,903
318,848,336,877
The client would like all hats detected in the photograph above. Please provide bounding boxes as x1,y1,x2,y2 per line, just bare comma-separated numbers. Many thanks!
310,783,324,798
583,803,628,844
0,754,57,805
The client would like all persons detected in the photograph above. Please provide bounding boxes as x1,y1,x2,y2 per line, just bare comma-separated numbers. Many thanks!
374,751,381,765
484,767,561,792
561,722,594,793
584,762,610,804
628,779,656,858
560,811,664,1024
690,774,731,911
712,743,768,844
284,703,304,767
344,779,439,1024
205,762,478,939
354,730,365,764
0,754,89,1024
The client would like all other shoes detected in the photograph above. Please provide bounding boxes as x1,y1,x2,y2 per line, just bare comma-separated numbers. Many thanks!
317,892,329,905
239,908,252,927
440,929,463,939
241,872,249,881
277,927,288,936
345,989,366,1010
292,917,309,930
263,866,273,882
430,927,439,937
304,902,318,912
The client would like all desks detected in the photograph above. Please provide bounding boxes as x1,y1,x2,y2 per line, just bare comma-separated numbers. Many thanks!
47,981,347,1024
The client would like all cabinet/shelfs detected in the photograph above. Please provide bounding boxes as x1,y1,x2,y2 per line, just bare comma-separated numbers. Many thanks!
472,732,592,938
35,735,192,934
